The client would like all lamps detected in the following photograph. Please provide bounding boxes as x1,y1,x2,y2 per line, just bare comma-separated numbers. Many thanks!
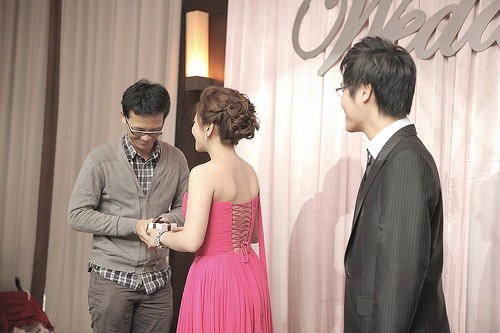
185,11,210,78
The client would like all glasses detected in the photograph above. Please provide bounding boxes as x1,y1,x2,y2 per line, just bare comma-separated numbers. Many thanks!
336,86,348,97
125,116,163,136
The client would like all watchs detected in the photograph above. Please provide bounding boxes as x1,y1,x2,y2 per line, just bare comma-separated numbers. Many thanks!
154,230,169,250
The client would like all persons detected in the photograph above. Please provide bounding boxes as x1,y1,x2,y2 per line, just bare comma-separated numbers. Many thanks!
146,85,275,333
67,79,190,332
338,35,452,332
